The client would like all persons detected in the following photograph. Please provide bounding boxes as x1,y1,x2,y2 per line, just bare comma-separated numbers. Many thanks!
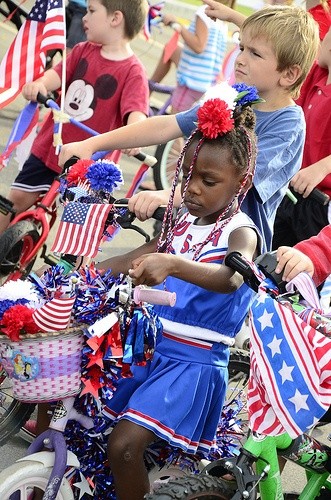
0,0,331,474
36,81,268,500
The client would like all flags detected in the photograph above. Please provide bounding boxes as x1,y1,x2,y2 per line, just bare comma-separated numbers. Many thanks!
0,0,65,110
50,201,113,258
31,297,76,332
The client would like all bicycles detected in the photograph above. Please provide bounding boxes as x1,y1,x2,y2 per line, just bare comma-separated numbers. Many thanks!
0,261,331,500
0,87,179,289
